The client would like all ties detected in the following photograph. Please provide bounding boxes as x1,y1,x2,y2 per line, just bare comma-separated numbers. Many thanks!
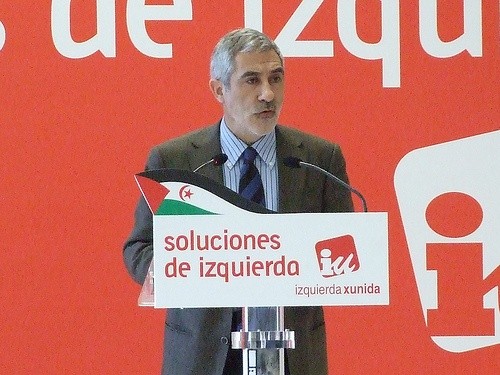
237,147,267,209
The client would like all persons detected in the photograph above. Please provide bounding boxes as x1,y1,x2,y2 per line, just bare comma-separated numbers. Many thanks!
121,28,355,375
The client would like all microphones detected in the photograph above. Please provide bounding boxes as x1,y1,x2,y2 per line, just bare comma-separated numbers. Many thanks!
282,156,367,212
191,152,228,176
